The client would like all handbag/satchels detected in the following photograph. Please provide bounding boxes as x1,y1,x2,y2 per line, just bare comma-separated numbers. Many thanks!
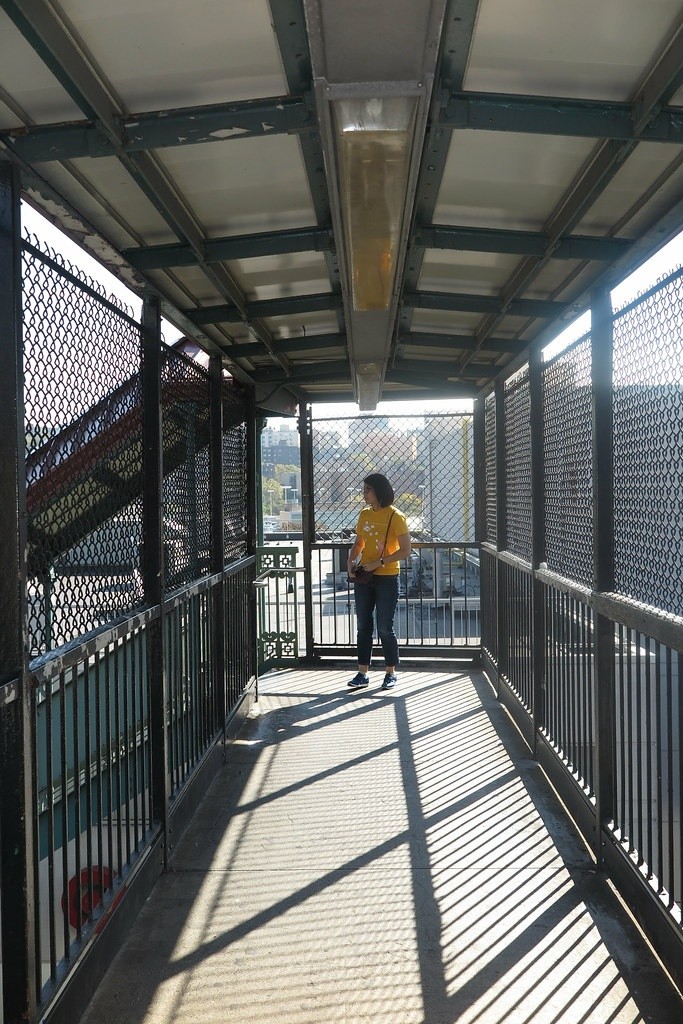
346,566,374,585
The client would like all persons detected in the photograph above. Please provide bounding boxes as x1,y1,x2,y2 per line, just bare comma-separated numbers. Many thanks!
347,474,411,690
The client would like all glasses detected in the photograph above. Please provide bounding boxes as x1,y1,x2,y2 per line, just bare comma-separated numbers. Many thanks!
363,486,374,493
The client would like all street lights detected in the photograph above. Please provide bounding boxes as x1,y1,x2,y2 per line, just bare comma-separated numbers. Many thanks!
267,490,274,516
345,487,354,510
282,486,291,503
356,488,361,501
291,489,298,504
419,485,426,520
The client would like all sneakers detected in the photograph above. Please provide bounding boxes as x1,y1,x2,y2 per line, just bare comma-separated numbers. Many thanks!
347,672,369,687
381,672,397,689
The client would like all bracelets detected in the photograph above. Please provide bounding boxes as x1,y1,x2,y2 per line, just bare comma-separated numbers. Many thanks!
379,558,385,568
